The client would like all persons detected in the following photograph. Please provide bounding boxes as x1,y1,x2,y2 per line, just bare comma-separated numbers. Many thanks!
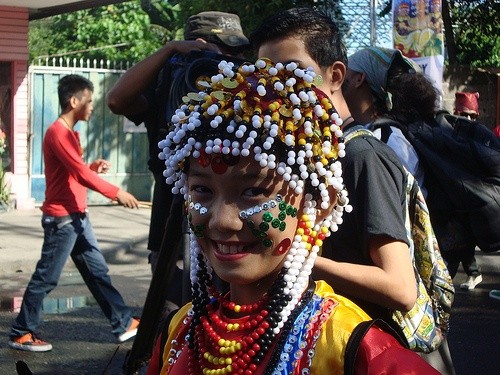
145,59,442,375
8,74,138,352
404,110,500,276
342,47,430,206
453,93,484,290
249,8,454,375
106,11,251,375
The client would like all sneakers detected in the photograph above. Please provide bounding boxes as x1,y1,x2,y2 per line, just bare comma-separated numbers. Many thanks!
117,318,138,342
461,274,483,291
8,334,54,353
490,289,500,300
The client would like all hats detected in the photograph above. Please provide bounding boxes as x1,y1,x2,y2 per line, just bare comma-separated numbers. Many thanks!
185,13,252,50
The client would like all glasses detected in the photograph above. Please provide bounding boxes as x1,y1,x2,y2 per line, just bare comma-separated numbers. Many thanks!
459,112,477,119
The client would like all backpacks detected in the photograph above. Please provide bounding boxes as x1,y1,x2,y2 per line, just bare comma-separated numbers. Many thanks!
382,165,454,351
405,113,500,258
